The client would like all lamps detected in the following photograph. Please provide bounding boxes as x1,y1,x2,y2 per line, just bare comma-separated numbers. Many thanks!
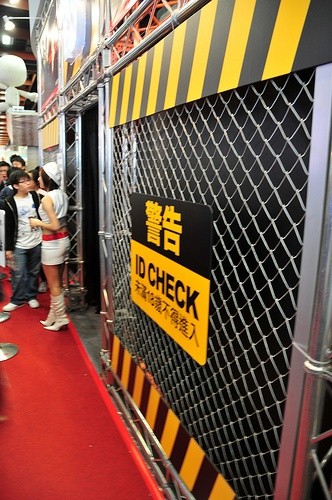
3,14,40,31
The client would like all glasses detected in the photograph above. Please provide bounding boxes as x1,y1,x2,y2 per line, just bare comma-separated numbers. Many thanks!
18,178,32,185
0,169,7,173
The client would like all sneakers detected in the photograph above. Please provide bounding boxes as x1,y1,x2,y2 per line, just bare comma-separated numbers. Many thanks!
28,299,39,308
2,302,25,311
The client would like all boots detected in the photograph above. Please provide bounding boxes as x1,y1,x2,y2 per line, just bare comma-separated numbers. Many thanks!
44,293,70,331
39,295,56,327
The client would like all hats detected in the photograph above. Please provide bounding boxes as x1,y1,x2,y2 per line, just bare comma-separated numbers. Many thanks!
42,161,61,187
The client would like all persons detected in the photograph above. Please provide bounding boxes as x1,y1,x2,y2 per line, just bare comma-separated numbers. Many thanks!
28,162,69,331
0,153,66,312
